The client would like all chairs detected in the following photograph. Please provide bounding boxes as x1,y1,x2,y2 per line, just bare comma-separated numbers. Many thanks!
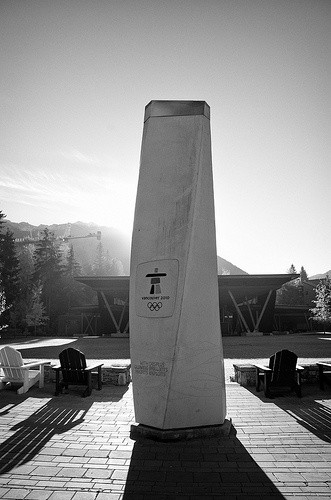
52,347,103,398
253,348,304,399
0,347,50,394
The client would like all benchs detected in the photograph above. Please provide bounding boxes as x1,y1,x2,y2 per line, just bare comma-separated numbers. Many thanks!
233,363,256,386
101,364,131,385
